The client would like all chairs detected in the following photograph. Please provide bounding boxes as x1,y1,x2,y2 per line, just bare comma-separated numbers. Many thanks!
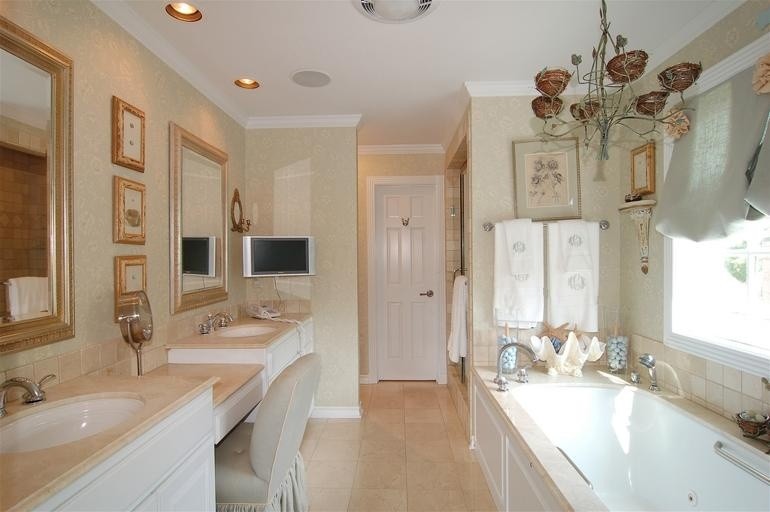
214,351,322,512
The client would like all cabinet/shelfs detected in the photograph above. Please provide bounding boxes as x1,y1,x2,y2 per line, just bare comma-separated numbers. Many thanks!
267,320,315,422
618,199,656,276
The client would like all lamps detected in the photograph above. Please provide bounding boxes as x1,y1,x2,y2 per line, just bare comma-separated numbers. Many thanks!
530,0,702,162
348,0,440,23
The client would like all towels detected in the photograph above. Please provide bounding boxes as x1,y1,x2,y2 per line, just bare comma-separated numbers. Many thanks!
4,276,49,321
546,219,600,334
446,274,467,363
493,217,545,331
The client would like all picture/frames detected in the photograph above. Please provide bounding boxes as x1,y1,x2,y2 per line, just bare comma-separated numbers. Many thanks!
512,136,582,220
630,141,655,195
113,255,148,324
110,94,147,174
112,174,147,246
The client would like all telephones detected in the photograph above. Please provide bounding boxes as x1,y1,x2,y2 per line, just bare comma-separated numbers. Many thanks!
246,304,281,319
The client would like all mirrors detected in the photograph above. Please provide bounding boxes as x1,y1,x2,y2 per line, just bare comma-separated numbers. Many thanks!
168,120,230,315
230,187,250,233
0,14,76,356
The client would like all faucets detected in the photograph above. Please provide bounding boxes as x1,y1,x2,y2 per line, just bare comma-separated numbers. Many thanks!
213,312,233,329
637,354,662,392
493,342,539,384
1,376,44,417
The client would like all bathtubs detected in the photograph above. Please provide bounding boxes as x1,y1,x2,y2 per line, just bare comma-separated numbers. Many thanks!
504,381,770,512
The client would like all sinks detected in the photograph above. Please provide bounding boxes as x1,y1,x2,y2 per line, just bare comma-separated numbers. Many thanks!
1,391,146,453
217,323,277,337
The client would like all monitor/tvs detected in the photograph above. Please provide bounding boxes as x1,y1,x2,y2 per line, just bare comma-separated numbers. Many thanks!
240,233,315,279
183,235,217,279
